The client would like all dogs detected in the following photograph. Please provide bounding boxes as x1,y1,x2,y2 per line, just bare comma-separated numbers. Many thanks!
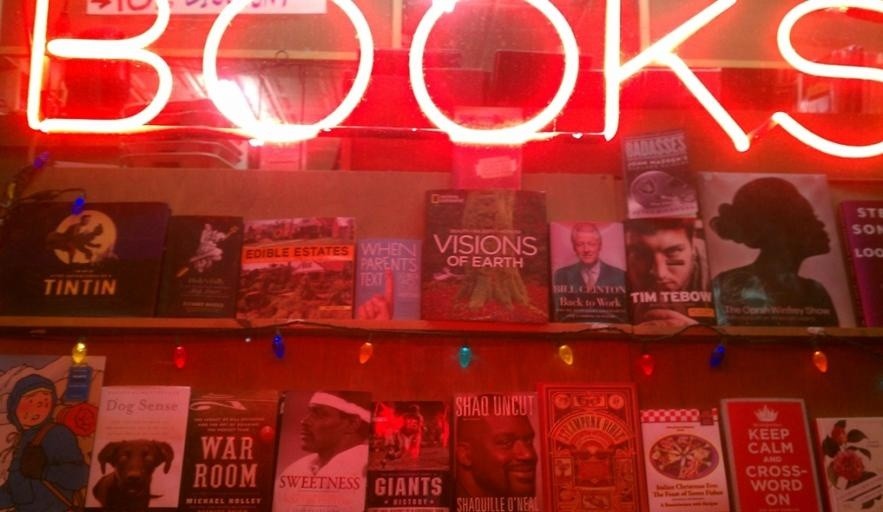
91,438,175,510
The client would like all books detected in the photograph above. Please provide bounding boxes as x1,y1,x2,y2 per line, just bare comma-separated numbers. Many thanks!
1,355,882,512
453,107,522,190
231,134,346,172
620,128,699,220
5,170,882,328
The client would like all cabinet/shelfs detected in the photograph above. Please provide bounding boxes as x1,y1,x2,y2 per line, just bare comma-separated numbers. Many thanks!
0,160,883,512
